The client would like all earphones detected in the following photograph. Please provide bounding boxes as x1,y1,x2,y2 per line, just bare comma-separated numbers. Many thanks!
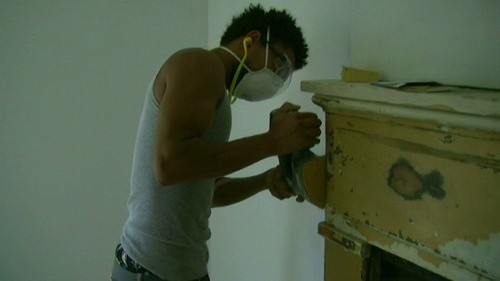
242,36,253,52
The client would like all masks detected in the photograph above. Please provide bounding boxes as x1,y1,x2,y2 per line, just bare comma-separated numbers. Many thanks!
218,25,284,103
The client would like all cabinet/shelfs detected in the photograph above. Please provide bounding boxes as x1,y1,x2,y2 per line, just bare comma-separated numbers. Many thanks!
298,77,500,281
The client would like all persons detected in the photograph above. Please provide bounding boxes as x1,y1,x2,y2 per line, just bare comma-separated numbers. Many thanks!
111,2,322,281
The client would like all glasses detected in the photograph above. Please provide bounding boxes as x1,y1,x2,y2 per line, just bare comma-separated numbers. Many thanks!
263,38,291,83
